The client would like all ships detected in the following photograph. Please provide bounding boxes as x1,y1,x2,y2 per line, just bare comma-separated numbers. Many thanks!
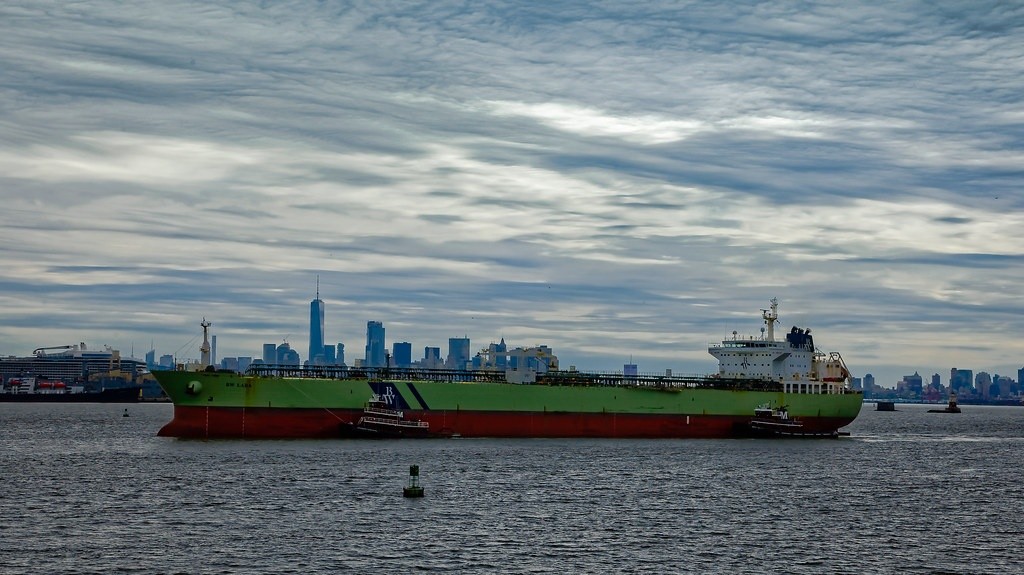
148,272,864,443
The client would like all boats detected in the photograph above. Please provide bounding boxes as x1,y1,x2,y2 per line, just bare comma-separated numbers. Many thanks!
749,400,806,436
872,400,899,412
926,392,962,415
346,374,430,439
